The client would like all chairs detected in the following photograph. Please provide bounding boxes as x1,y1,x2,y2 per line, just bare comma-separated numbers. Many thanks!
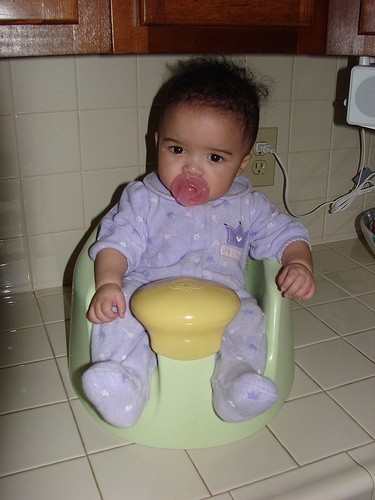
68,224,295,449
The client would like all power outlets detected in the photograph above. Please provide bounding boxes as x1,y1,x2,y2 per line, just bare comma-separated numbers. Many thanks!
241,127,278,187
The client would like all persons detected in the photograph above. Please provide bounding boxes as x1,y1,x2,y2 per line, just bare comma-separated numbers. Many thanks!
80,51,315,429
